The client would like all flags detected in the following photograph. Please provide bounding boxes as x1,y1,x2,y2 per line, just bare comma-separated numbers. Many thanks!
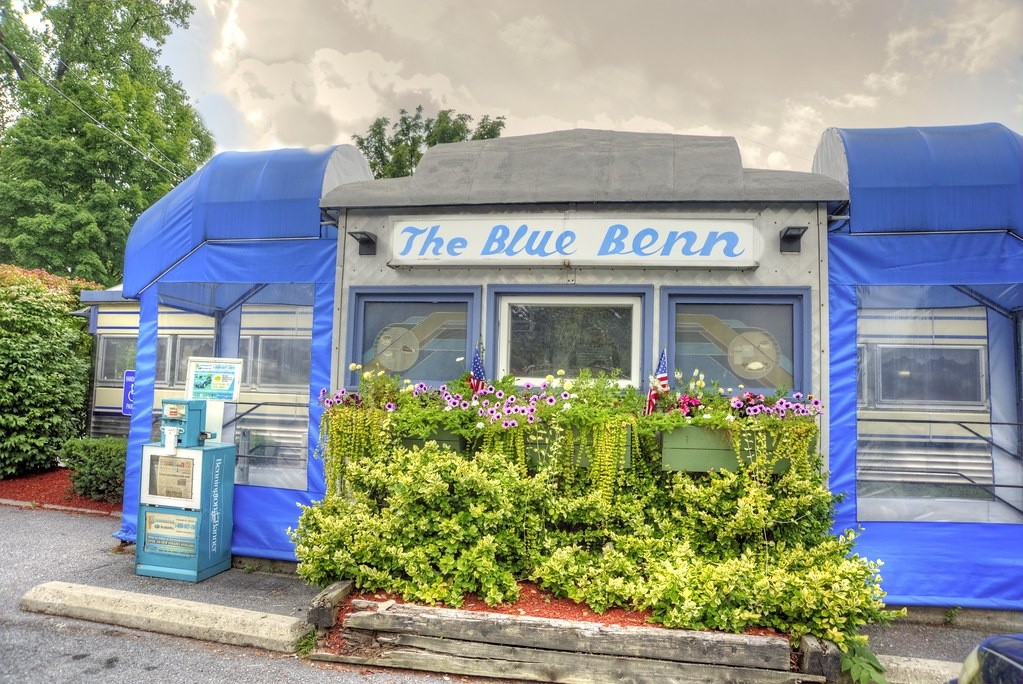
643,349,671,418
468,345,488,396
